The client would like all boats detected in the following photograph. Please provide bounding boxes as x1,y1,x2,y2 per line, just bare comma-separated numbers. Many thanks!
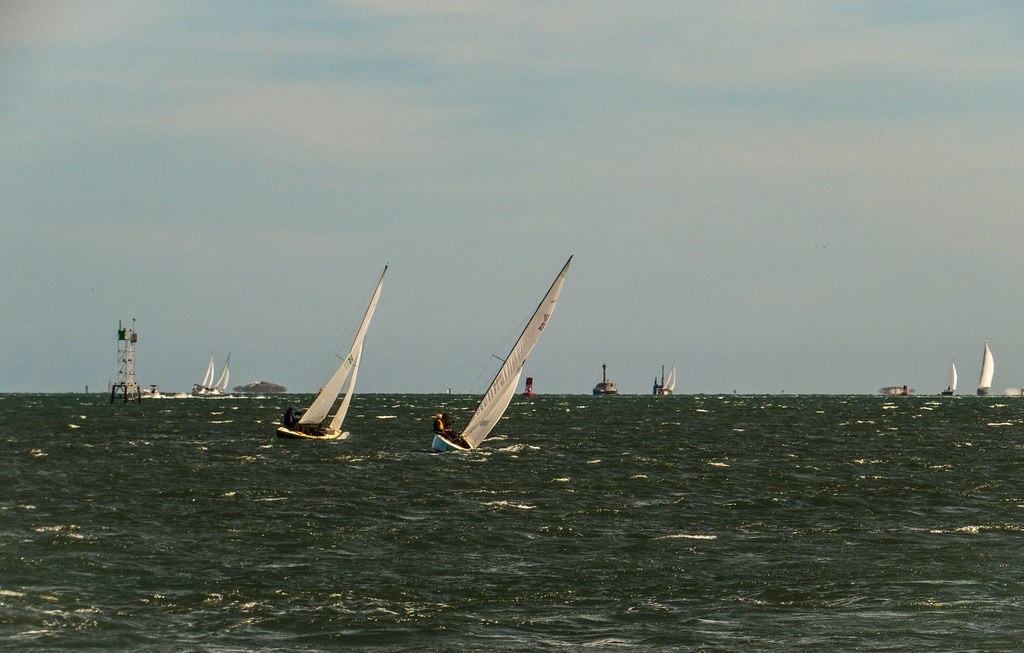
150,385,159,397
593,363,617,395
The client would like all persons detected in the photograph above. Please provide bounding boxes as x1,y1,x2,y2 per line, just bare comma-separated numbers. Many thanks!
433,411,459,442
285,406,304,430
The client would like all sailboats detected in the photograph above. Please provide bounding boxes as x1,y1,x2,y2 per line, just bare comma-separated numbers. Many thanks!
277,266,388,439
192,347,231,397
940,358,958,395
654,361,676,395
977,339,995,397
429,254,573,452
